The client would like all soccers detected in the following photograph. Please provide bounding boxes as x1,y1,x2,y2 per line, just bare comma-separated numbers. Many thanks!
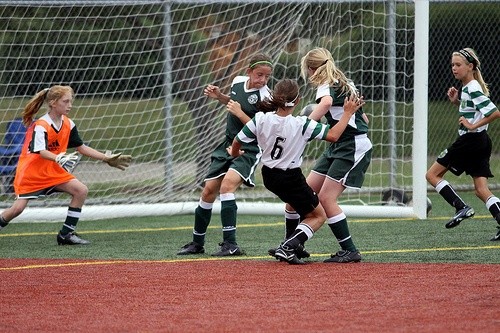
299,104,329,126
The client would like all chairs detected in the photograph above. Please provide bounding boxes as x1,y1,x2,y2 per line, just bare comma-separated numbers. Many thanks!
0,117,37,196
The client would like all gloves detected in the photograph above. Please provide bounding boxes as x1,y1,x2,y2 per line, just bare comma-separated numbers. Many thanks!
55,151,78,172
103,153,131,171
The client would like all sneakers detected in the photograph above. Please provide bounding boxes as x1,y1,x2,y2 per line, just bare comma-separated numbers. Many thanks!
56,230,90,245
445,204,475,229
489,226,500,241
176,241,206,255
275,244,305,264
323,250,361,263
211,241,241,256
268,249,311,259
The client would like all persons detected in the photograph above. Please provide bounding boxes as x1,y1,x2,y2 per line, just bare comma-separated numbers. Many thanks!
177,54,275,257
225,79,366,265
0,85,135,244
424,47,500,242
267,47,373,263
176,15,261,189
